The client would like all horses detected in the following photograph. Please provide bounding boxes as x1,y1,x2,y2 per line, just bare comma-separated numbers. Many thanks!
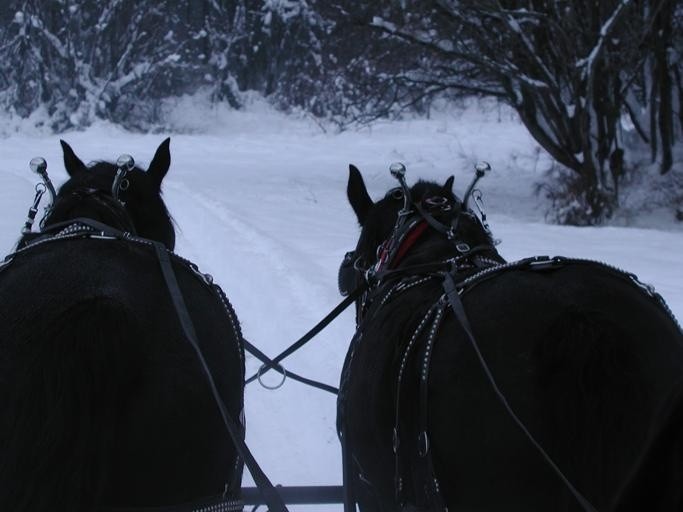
0,137,245,511
336,163,682,511
